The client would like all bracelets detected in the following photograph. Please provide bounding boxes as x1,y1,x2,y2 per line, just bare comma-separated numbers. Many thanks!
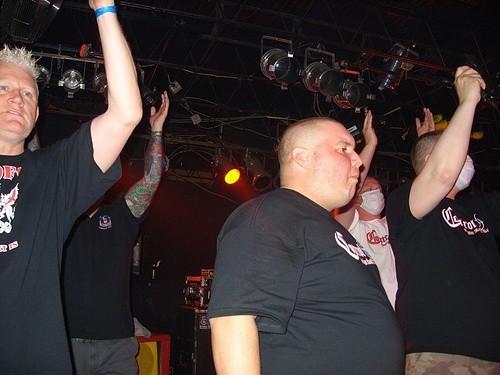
95,5,118,16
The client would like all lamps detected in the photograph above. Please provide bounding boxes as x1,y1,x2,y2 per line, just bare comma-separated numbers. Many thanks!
36,54,53,89
258,35,307,91
92,61,108,106
240,153,273,192
58,56,87,99
209,144,242,187
136,66,157,109
368,41,420,94
323,60,377,114
301,42,349,103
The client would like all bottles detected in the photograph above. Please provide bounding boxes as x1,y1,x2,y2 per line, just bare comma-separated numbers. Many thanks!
199,275,209,308
470,63,497,103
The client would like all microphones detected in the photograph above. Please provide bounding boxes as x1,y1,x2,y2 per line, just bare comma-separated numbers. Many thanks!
481,90,495,104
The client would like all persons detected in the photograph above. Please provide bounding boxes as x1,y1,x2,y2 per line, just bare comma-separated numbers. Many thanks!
0,0,142,375
24,65,500,375
